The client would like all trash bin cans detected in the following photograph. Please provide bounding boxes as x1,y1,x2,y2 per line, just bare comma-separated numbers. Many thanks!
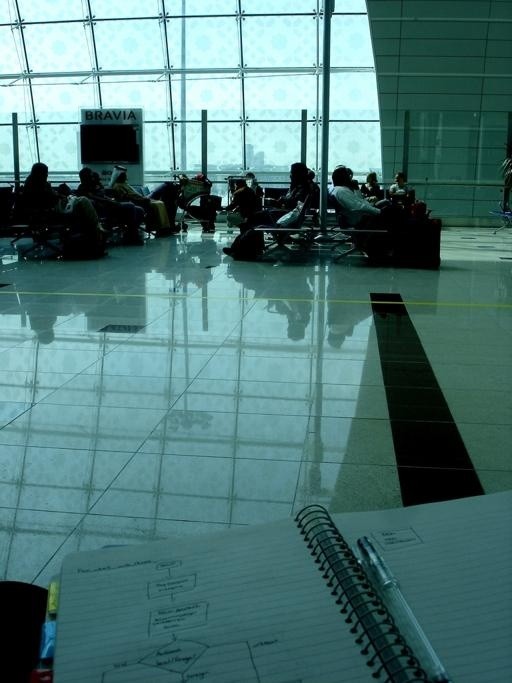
264,187,289,207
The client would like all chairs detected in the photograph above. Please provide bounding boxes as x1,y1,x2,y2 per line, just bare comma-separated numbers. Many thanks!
0,175,442,271
489,200,512,235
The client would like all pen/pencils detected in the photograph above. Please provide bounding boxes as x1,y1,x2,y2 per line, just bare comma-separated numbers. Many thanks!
357,536,451,683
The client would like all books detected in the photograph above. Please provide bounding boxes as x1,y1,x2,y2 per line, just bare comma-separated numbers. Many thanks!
51,487,510,682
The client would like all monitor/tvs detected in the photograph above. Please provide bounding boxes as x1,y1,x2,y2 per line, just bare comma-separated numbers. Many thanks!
80,124,142,165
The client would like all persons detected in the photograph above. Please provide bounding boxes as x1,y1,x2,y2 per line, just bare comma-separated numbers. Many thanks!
18,163,181,261
222,162,412,264
